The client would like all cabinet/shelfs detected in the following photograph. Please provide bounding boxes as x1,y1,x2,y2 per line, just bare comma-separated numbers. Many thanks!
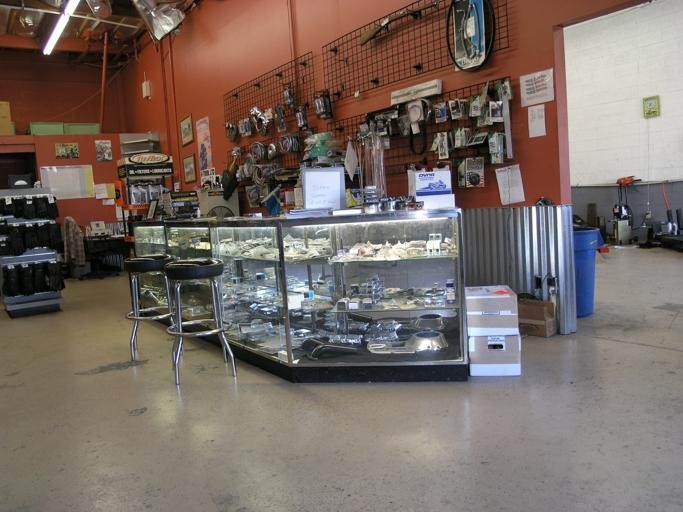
0,186,64,318
128,204,468,383
116,152,175,249
120,132,160,158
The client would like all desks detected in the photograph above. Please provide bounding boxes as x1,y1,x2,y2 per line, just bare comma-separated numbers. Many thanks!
83,235,125,272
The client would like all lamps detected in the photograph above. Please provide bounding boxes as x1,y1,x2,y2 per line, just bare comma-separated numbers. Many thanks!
41,11,71,57
62,0,80,17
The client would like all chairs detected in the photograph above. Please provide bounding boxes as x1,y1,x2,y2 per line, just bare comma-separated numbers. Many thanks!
89,234,121,279
64,215,99,280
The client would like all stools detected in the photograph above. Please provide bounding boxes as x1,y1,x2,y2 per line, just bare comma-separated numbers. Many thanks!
165,256,236,385
123,253,183,361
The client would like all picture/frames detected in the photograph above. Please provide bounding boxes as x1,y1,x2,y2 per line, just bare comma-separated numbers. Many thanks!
179,112,194,147
182,152,197,184
642,95,660,118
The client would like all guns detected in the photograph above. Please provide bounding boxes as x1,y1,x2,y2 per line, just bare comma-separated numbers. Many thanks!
360,0,443,46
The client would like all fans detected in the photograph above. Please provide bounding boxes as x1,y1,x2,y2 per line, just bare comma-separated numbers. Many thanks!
206,205,234,216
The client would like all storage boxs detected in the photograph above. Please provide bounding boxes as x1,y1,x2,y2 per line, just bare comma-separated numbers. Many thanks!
468,332,522,377
518,299,558,338
64,122,100,135
0,100,13,136
464,285,518,336
26,122,64,135
